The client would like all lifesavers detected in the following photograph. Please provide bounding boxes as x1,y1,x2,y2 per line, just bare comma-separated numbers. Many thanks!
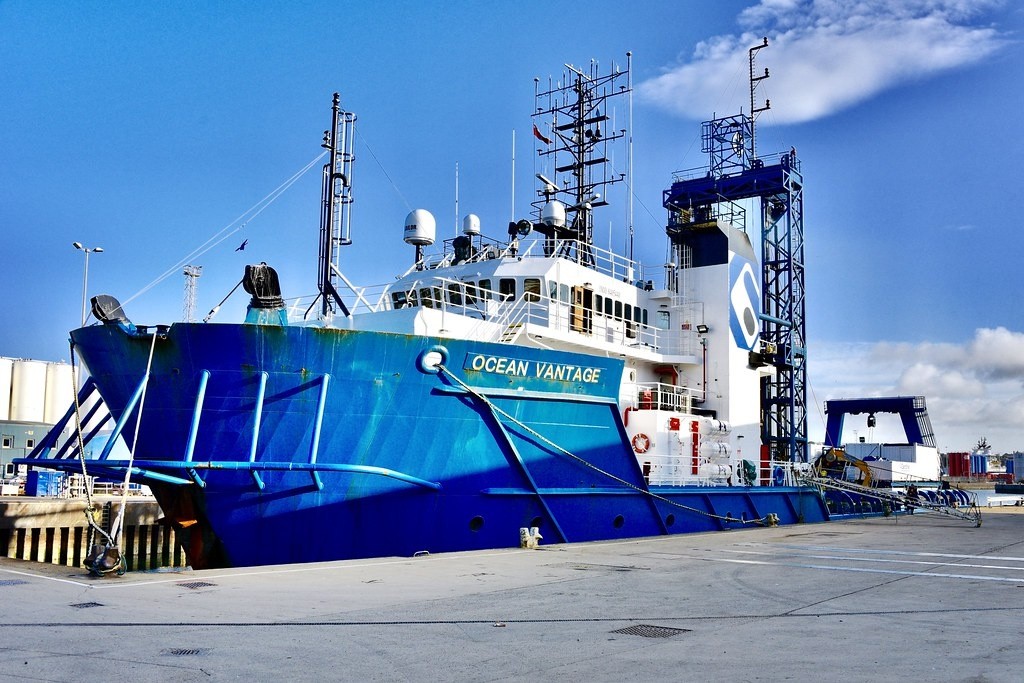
773,466,784,485
631,433,650,454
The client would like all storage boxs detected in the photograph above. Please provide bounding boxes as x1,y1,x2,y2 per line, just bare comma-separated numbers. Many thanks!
25,470,66,497
940,452,987,478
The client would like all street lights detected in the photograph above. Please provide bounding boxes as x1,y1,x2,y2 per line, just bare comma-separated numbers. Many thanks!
73,242,103,393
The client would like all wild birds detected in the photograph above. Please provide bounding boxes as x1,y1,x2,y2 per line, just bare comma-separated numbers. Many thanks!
235,239,248,251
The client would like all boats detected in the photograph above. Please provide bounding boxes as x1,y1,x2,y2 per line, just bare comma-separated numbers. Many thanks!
69,37,974,570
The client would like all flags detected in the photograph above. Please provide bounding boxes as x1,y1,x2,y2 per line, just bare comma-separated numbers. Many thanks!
532,124,554,144
